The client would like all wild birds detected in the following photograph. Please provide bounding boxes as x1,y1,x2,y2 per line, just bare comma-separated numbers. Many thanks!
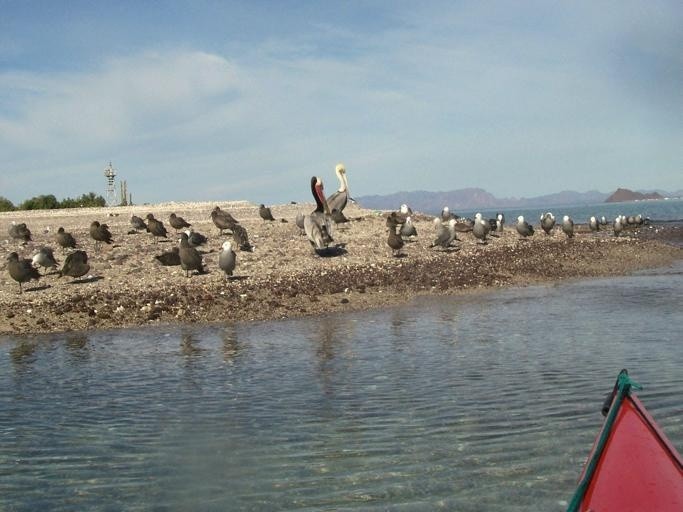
331,207,350,230
516,212,574,240
295,213,306,237
590,214,650,235
304,177,334,251
326,164,349,213
258,203,275,224
384,203,505,259
7,220,116,294
128,206,253,277
